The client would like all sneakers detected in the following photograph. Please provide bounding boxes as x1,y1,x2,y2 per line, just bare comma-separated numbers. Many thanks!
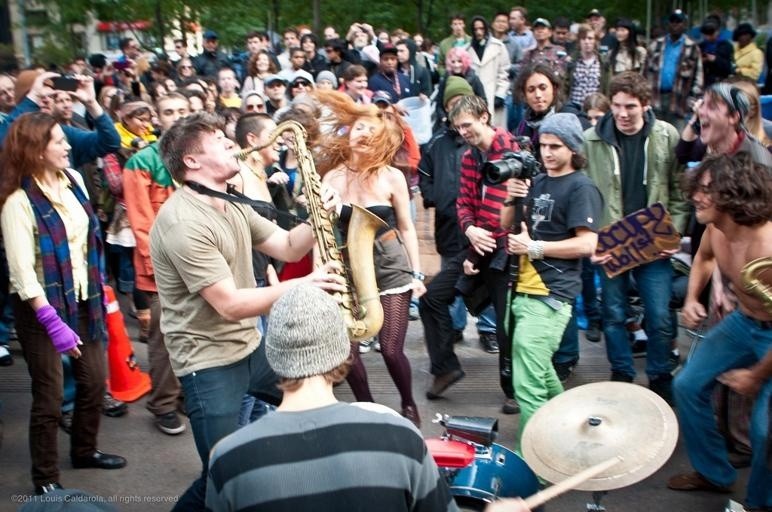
503,398,519,416
585,320,601,342
57,410,72,432
479,331,501,355
374,340,381,352
70,450,126,469
101,392,128,416
667,470,728,491
0,345,14,367
401,404,421,428
449,331,464,346
648,374,676,408
426,370,465,400
608,371,631,382
34,481,63,496
358,342,371,354
154,413,186,436
552,356,579,383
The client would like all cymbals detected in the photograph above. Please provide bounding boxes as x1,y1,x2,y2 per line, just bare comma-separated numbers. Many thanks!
520,381,680,492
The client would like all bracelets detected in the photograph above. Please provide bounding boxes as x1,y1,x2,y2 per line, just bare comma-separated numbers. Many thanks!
411,270,426,281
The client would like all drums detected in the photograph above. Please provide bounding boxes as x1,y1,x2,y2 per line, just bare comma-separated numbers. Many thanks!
438,436,542,512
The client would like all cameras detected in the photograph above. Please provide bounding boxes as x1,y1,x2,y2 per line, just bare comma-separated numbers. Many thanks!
481,138,541,186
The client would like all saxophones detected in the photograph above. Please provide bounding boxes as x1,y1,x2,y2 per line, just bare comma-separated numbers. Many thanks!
232,119,391,344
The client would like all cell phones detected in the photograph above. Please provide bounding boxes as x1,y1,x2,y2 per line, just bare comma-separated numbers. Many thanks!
50,77,81,92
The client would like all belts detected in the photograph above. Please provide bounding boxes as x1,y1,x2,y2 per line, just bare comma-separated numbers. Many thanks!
514,289,578,311
740,313,772,330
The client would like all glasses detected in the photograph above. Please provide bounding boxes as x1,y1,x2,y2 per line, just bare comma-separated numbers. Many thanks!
292,80,308,87
248,105,264,111
182,65,193,70
132,115,151,124
177,45,182,49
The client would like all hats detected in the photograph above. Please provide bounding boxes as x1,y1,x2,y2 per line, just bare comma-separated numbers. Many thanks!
531,17,551,29
540,112,583,156
712,84,754,141
262,77,281,83
379,43,398,55
669,9,688,21
315,69,339,88
13,70,55,105
373,91,392,105
264,286,347,378
701,18,720,33
731,21,756,41
586,9,601,18
202,31,217,40
442,75,475,106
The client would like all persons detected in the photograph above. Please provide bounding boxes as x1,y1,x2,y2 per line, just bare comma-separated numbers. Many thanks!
434,46,489,128
226,113,286,430
418,95,529,414
0,72,17,367
698,15,734,89
499,112,605,486
670,150,772,512
122,91,213,435
648,9,704,132
114,38,195,110
0,112,128,496
583,71,693,404
610,19,647,77
583,93,608,127
149,111,347,512
195,25,307,111
566,24,610,113
104,100,158,319
469,15,511,135
436,13,472,78
205,280,460,512
306,87,427,431
490,11,524,106
731,22,765,85
551,16,573,49
416,76,503,356
524,16,571,93
307,20,434,96
509,6,536,54
51,90,104,222
584,8,619,60
0,70,131,434
515,61,604,344
676,77,771,245
65,52,114,107
689,82,772,469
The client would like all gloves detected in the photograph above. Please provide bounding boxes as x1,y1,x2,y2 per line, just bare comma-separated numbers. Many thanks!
33,304,80,353
